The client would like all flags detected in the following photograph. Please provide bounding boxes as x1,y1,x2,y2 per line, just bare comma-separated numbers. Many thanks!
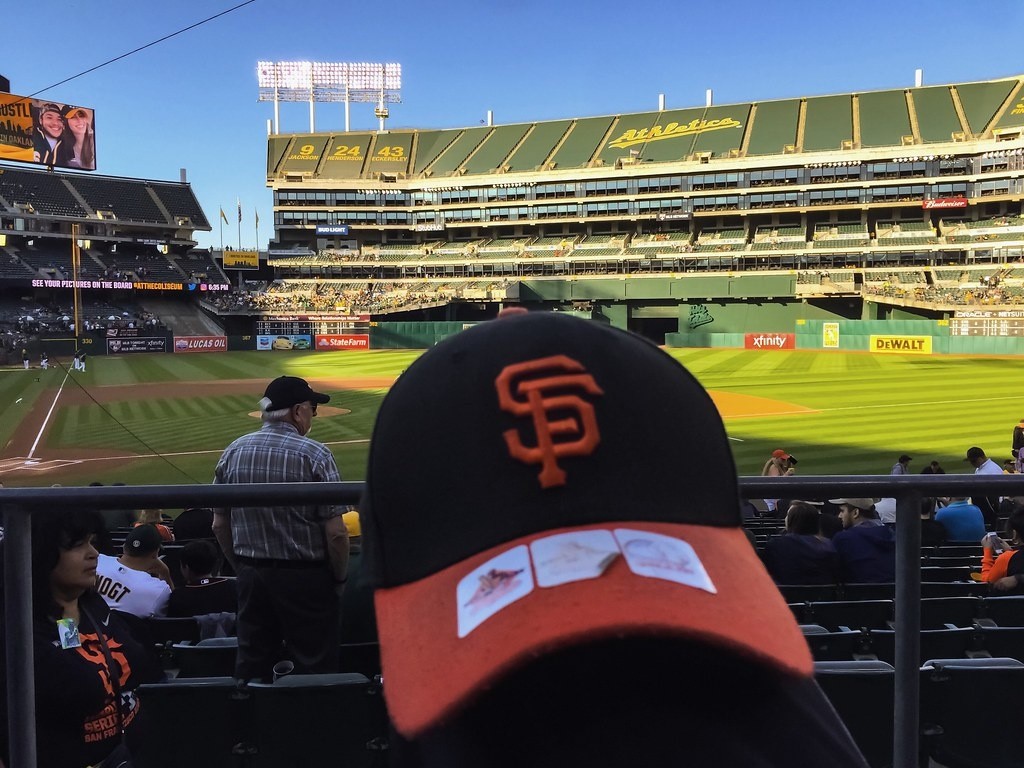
256,212,259,228
238,204,241,222
221,210,229,224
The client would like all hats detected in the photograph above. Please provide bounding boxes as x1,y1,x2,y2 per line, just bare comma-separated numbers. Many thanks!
263,375,331,411
124,525,163,555
771,449,791,461
962,446,983,461
362,310,815,739
829,498,875,510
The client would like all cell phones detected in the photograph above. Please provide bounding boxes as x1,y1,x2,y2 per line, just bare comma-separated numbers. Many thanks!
988,532,1002,555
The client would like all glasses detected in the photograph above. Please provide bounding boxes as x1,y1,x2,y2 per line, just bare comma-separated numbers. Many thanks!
301,403,318,413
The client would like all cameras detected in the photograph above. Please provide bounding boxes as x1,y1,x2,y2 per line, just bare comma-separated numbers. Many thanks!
788,456,798,465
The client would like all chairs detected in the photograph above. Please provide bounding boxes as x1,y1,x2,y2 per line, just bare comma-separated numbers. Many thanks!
104,513,1024,767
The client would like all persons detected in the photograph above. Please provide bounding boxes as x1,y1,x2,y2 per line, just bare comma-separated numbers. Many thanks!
78,353,87,372
0,481,390,768
73,350,80,370
742,420,1024,768
33,102,95,169
230,246,232,251
203,279,512,311
210,245,213,254
40,352,50,370
0,250,226,351
225,245,229,251
276,164,1024,306
212,375,351,684
22,349,29,369
